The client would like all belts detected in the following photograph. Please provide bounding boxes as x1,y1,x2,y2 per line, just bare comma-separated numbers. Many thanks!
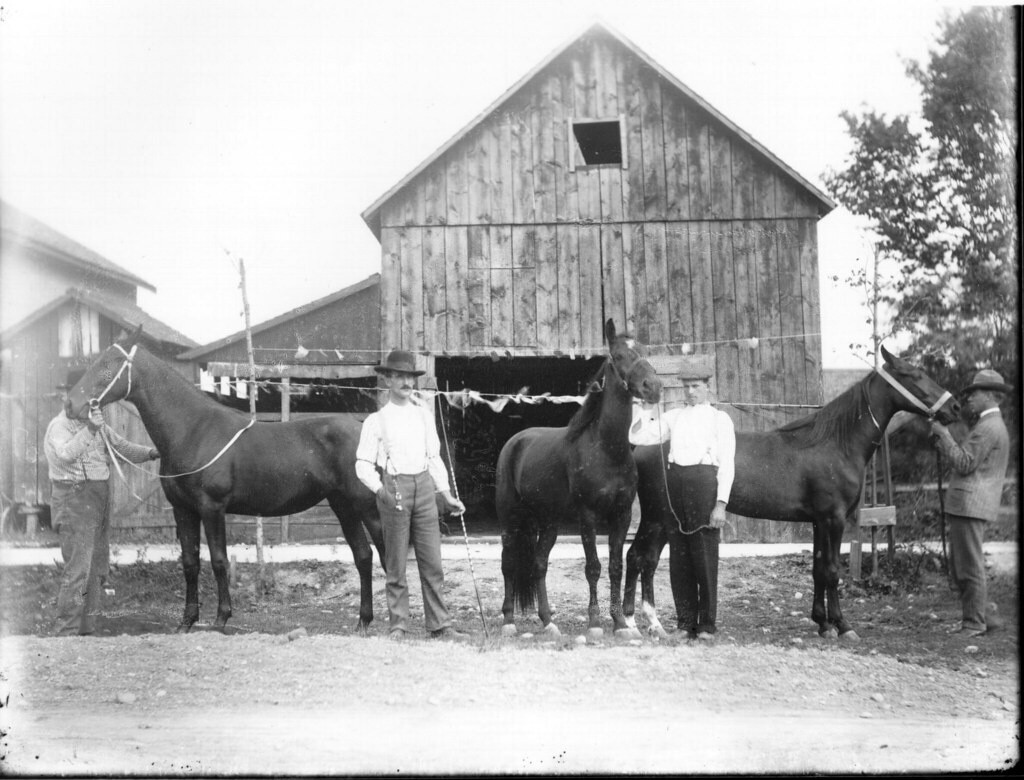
53,481,87,489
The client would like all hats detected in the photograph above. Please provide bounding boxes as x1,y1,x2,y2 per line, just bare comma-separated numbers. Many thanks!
677,362,712,380
374,352,426,376
960,370,1013,395
56,366,90,389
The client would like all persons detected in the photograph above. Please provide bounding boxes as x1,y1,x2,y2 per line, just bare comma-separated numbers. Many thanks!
44,370,162,638
628,363,736,642
356,350,470,640
930,370,1014,637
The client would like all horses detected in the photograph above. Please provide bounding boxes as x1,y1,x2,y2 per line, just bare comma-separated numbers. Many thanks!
621,344,963,645
63,322,387,635
495,319,662,640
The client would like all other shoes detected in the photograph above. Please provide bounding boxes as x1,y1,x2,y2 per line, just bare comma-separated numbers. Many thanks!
431,628,470,644
947,626,985,638
668,629,693,641
389,629,406,640
698,631,714,640
